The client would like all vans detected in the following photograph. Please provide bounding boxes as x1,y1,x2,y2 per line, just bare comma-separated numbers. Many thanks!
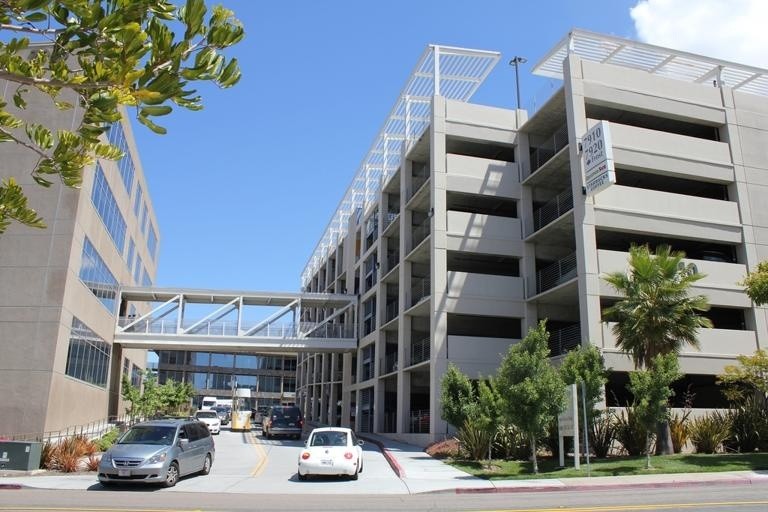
96,414,218,486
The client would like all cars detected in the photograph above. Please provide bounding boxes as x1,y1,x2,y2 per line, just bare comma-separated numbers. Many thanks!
412,409,429,431
294,424,366,482
193,396,303,440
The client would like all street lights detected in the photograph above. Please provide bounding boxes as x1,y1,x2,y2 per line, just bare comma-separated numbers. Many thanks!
509,55,527,107
